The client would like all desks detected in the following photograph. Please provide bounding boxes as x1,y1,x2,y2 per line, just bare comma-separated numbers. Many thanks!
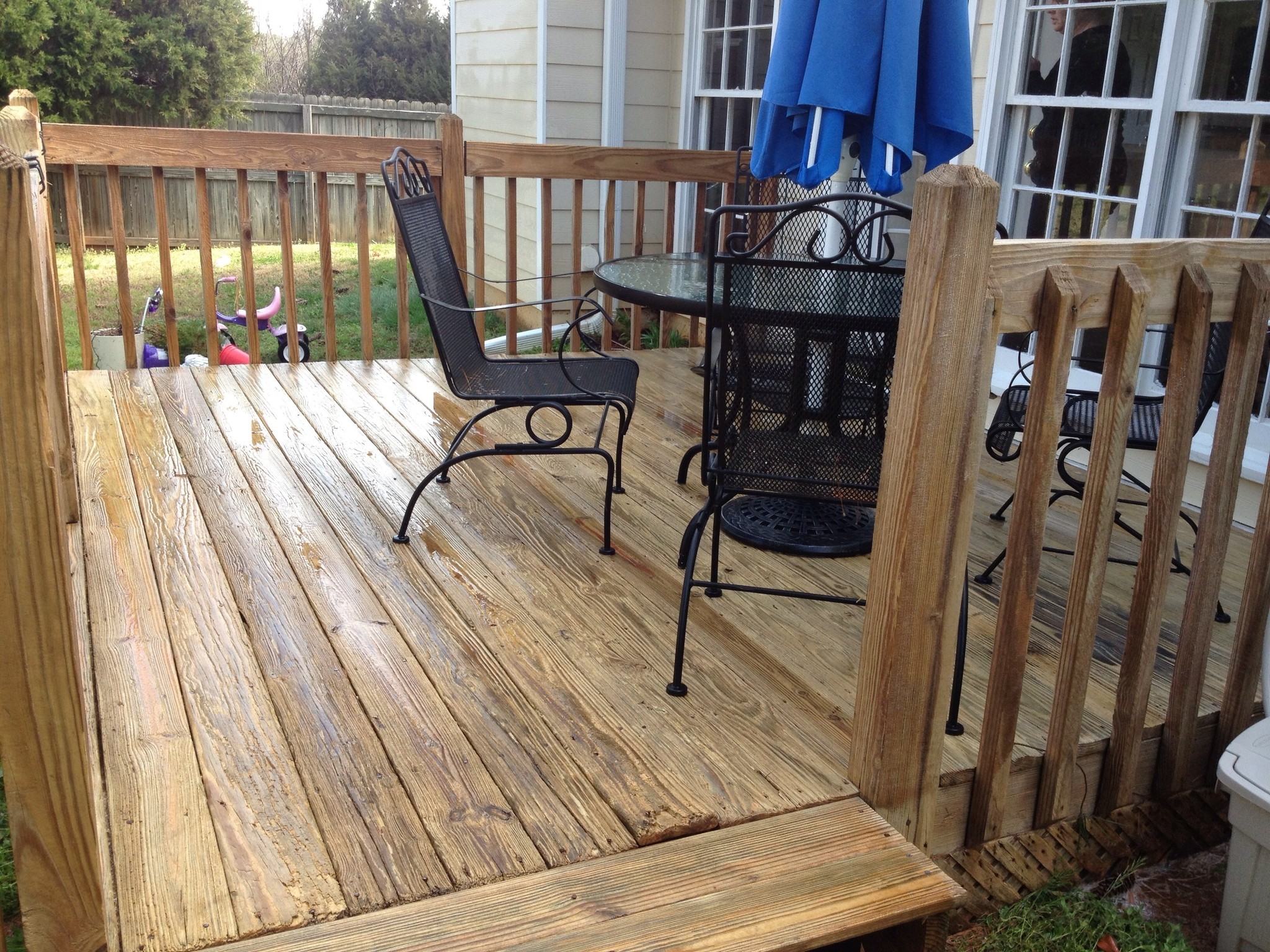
594,252,902,571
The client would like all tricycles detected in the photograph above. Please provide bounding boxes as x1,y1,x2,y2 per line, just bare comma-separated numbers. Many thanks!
202,275,310,363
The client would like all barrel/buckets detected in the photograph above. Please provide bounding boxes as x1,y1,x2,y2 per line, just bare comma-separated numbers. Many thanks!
220,344,250,365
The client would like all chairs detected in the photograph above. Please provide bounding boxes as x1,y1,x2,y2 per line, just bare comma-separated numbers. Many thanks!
380,127,1270,734
